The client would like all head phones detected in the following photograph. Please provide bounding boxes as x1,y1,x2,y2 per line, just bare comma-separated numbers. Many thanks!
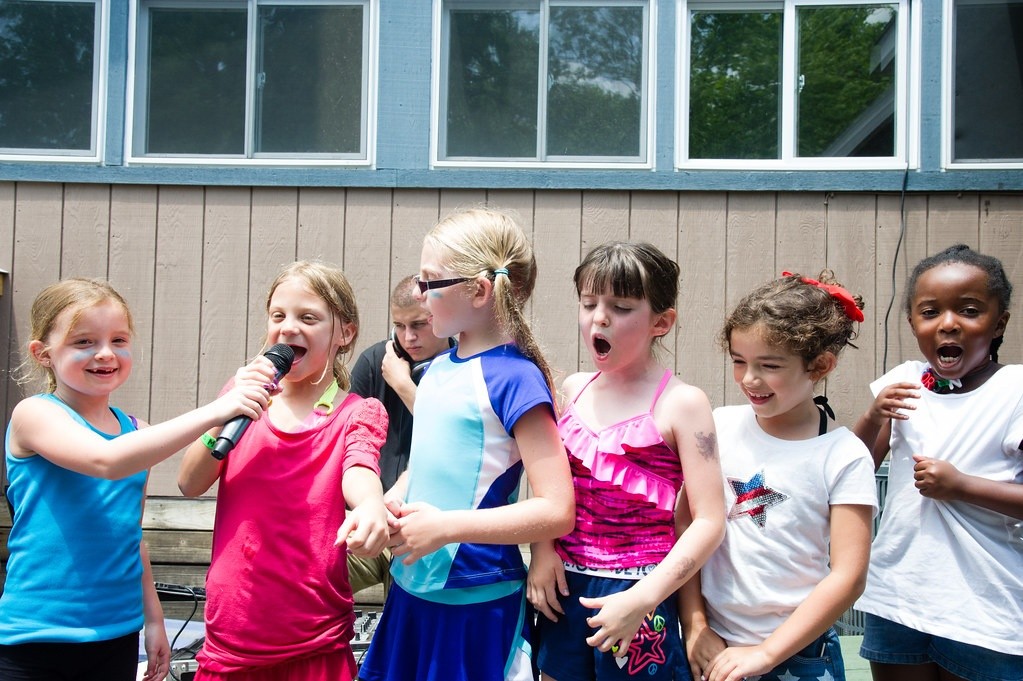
390,325,404,357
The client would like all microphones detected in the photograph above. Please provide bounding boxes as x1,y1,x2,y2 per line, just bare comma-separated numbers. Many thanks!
210,343,295,461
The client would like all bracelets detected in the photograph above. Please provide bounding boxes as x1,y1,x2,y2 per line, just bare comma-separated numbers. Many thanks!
200,433,217,450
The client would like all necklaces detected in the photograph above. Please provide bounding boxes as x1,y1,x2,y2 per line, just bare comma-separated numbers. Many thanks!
920,361,992,395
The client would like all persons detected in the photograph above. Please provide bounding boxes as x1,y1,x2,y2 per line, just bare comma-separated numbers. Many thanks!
676,270,878,681
526,240,726,681
178,262,389,681
357,209,576,681
342,274,459,606
1,278,271,680
853,243,1023,681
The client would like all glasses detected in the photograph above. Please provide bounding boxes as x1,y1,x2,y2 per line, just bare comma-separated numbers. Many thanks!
412,275,496,298
